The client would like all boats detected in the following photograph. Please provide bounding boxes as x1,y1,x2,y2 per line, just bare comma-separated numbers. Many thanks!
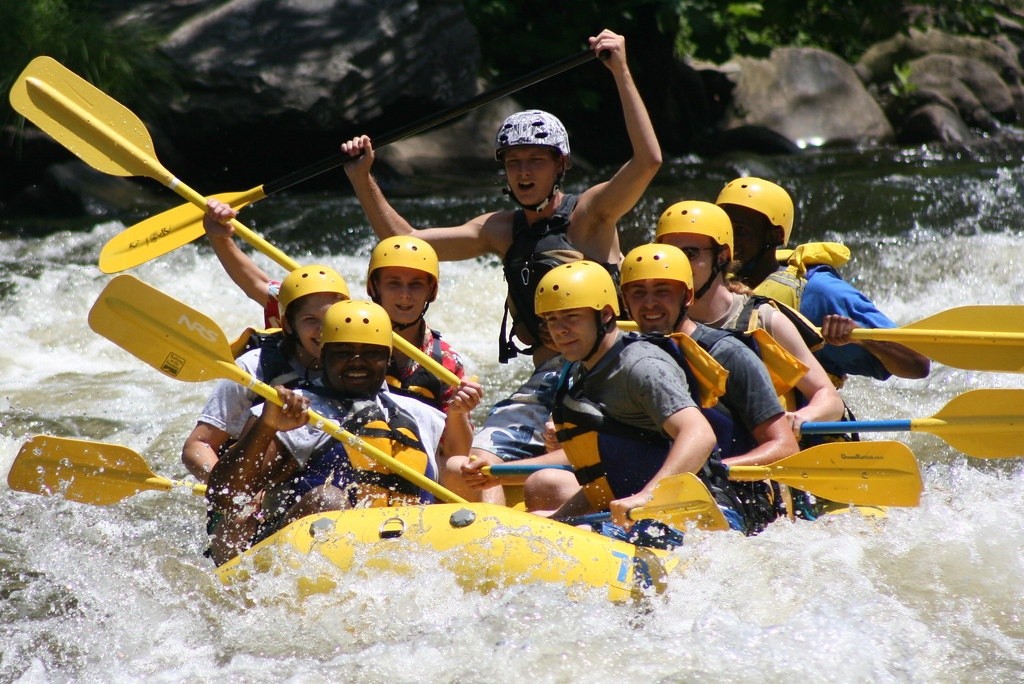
212,499,890,609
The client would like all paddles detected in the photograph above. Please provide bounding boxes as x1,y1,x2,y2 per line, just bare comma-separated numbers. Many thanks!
87,273,468,502
7,54,463,387
617,304,1024,374
97,49,610,274
797,389,1024,459
471,441,926,510
559,471,729,535
6,433,207,507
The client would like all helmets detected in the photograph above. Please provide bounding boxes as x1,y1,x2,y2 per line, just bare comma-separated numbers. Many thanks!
494,109,570,162
655,200,734,270
535,261,623,317
714,176,793,249
279,265,351,336
366,236,440,304
318,299,394,367
618,243,695,308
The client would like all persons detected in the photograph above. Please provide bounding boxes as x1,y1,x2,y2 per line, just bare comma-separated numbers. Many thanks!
205,300,448,568
534,260,731,551
341,30,662,369
203,199,482,502
459,177,930,536
182,265,351,483
